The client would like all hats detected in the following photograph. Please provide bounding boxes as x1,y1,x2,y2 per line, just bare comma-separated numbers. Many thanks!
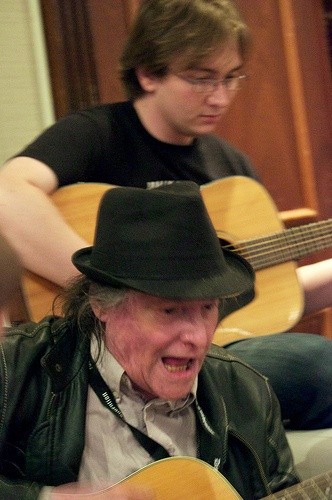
71,180,257,301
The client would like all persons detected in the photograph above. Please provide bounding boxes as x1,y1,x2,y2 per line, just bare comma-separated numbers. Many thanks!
0,1,332,429
0,180,301,500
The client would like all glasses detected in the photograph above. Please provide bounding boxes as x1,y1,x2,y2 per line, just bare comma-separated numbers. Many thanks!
171,69,248,93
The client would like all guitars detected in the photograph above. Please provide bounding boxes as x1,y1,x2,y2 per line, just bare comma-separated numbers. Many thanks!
17,174,332,352
88,455,332,500
